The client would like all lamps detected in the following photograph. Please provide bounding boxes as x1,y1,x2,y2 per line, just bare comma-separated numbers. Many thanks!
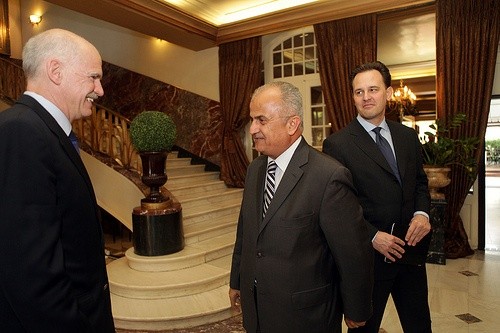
390,81,420,115
28,13,42,24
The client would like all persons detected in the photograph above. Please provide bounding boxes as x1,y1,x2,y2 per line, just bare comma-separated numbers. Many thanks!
322,62,432,333
229,81,373,333
0,29,116,333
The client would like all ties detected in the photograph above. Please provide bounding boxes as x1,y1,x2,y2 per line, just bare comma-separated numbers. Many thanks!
68,130,80,156
371,127,401,185
262,161,278,220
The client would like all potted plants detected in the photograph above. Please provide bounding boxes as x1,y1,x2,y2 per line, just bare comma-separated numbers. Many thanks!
412,112,480,201
127,110,177,203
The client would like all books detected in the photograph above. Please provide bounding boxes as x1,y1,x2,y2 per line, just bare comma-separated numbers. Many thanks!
385,223,432,267
